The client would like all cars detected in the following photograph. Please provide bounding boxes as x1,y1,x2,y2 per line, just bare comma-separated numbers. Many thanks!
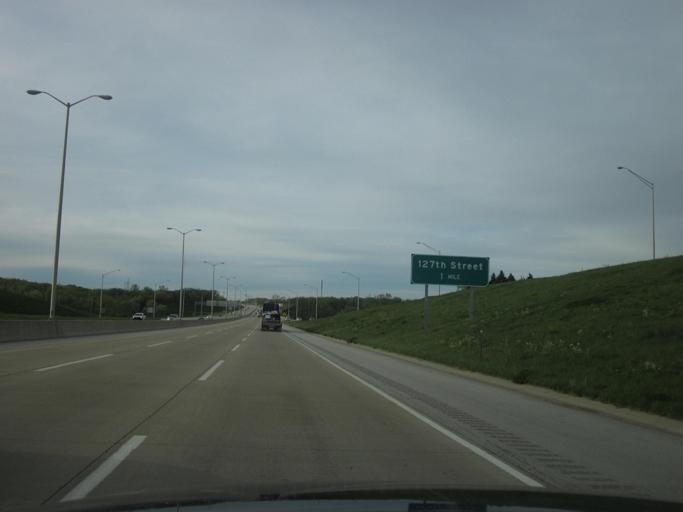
133,313,147,320
262,314,283,331
167,314,179,321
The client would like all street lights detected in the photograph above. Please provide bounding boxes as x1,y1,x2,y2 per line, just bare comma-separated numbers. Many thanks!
204,260,236,316
27,89,113,319
617,166,655,259
304,283,318,320
416,241,441,296
100,269,120,319
343,271,360,310
166,227,202,318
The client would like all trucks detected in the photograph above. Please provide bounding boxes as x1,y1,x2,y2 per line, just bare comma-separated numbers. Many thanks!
263,303,280,313
